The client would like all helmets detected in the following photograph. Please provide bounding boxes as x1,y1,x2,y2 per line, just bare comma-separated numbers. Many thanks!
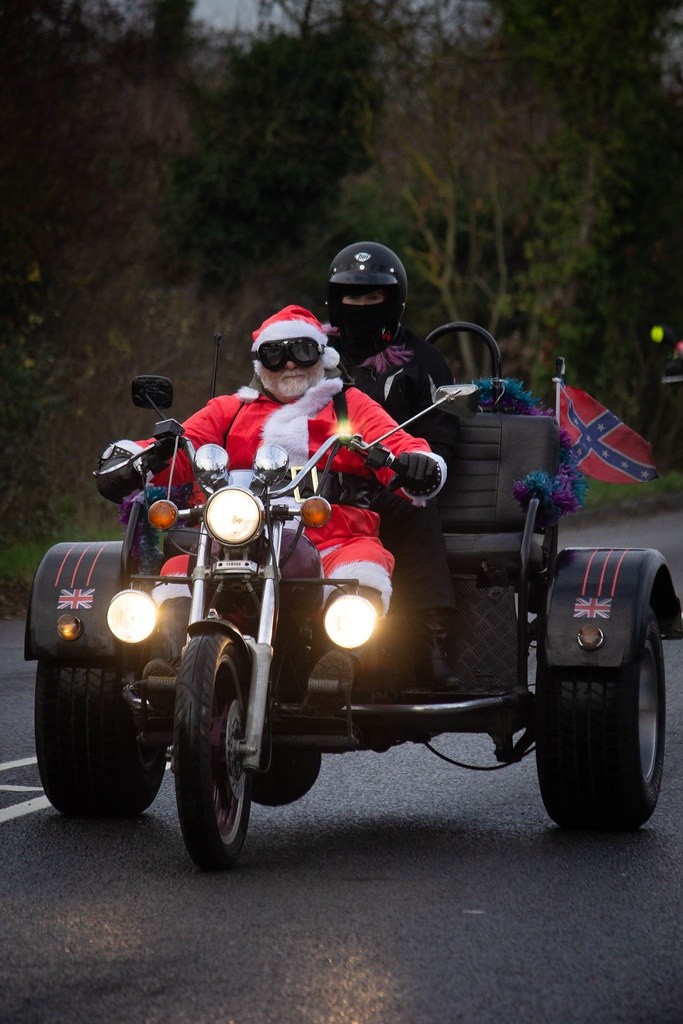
325,239,409,302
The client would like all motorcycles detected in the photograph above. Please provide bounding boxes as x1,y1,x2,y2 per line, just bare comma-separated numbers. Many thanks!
22,372,683,874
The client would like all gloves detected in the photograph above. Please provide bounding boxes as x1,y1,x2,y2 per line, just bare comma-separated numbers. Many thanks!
385,451,442,499
95,443,146,506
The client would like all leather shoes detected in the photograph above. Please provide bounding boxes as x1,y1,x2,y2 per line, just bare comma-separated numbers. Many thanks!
141,657,178,720
296,647,355,718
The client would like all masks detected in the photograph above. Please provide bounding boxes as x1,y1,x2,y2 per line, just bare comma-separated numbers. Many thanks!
340,300,395,338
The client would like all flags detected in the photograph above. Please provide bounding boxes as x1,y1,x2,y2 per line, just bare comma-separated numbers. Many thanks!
557,377,660,485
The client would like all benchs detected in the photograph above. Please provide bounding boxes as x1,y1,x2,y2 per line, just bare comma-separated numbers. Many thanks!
437,410,562,699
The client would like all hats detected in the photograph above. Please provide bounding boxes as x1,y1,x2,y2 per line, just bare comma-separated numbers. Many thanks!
250,302,340,371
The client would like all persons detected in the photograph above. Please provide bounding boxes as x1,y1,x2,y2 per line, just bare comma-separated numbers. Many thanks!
317,242,464,693
96,305,448,723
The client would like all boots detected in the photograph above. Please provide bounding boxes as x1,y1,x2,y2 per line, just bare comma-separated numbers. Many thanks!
415,609,461,690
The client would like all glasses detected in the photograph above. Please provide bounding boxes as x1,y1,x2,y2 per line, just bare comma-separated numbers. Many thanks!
249,336,328,371
